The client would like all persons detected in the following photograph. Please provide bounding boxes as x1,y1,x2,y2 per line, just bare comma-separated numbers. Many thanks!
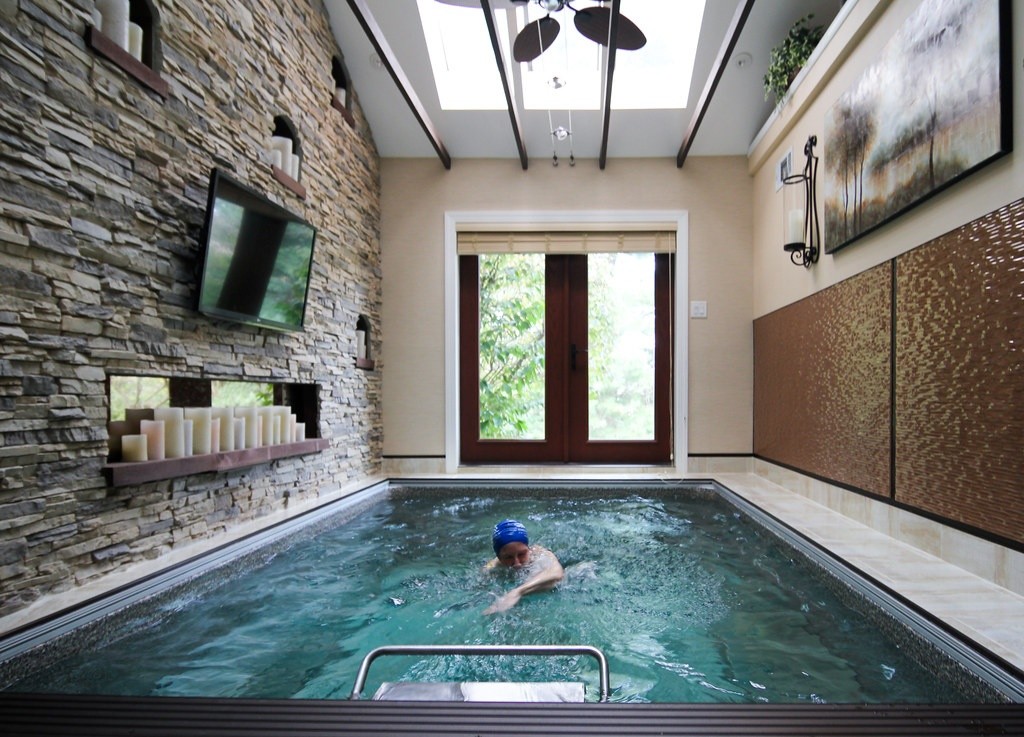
481,509,563,616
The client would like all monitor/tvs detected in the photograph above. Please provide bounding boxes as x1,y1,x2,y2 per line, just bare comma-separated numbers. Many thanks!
193,168,318,334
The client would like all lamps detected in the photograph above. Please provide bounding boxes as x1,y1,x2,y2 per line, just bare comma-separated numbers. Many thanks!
783,135,821,269
509,0,647,62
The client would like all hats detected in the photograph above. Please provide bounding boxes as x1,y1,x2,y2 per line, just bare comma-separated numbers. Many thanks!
492,520,529,556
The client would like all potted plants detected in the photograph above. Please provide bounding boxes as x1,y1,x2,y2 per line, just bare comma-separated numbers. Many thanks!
762,12,823,103
